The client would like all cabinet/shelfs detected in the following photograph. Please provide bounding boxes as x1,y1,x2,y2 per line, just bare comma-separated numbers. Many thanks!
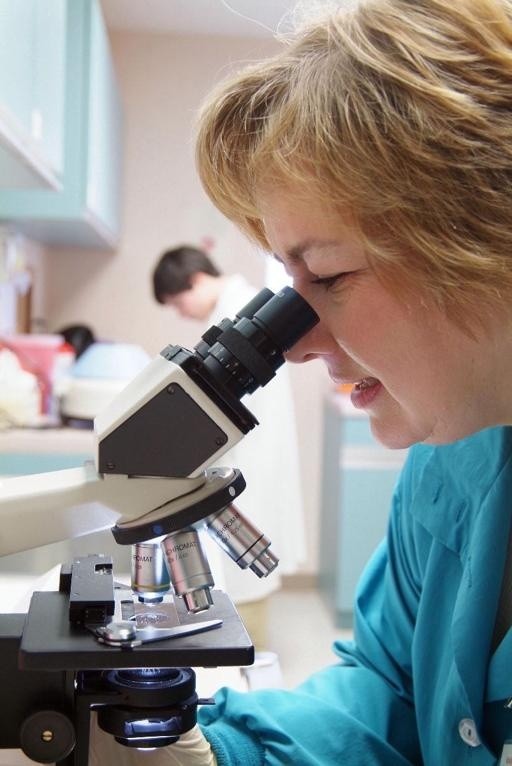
0,0,68,191
316,390,410,629
0,0,120,249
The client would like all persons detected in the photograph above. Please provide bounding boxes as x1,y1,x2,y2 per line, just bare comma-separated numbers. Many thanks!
86,0,512,765
154,246,308,694
55,323,151,404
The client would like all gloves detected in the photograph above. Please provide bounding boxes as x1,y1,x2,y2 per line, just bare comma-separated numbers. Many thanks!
87,713,216,766
